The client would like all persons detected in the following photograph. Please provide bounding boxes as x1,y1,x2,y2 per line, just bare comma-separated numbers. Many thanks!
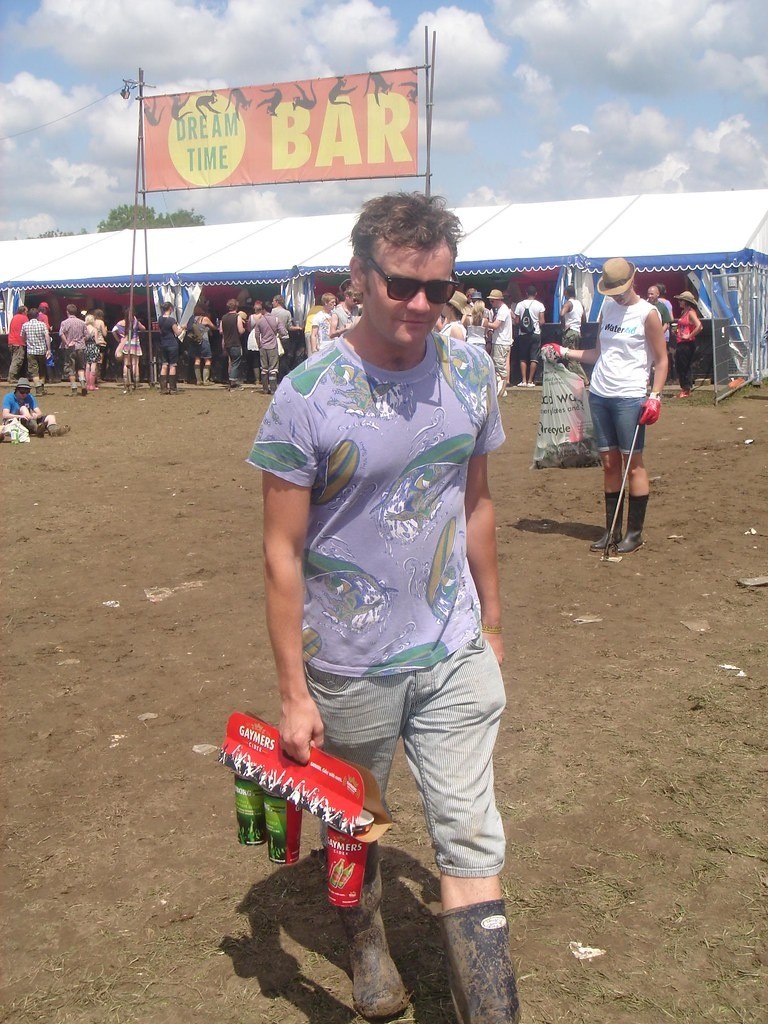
1,378,71,438
247,195,521,1024
645,284,703,399
542,260,668,554
436,285,587,397
7,281,361,396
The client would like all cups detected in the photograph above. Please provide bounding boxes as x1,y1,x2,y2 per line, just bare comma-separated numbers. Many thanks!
234,778,267,845
327,809,374,907
264,794,302,864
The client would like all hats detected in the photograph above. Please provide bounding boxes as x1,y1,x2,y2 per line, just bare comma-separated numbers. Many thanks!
596,258,634,296
15,377,32,389
39,301,50,312
674,291,695,306
448,290,468,314
470,292,482,299
486,289,504,300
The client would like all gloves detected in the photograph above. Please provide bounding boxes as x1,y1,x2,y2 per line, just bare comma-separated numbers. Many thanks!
541,343,569,359
640,391,661,425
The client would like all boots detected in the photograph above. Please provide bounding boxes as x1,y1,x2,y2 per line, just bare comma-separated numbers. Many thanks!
25,418,70,438
159,375,184,394
437,898,523,1024
35,384,47,397
260,374,277,394
590,489,649,556
196,365,215,385
323,839,407,1020
85,371,99,390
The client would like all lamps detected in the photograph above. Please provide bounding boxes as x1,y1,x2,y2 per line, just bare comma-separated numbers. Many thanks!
120,81,130,100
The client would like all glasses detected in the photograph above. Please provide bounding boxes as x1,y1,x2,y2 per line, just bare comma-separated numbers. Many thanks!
351,297,360,304
17,388,31,394
354,248,461,304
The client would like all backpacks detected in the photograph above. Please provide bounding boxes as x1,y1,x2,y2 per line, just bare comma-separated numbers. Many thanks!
186,316,205,345
519,299,536,336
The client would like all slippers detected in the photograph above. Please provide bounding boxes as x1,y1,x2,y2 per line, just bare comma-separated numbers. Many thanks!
584,383,589,387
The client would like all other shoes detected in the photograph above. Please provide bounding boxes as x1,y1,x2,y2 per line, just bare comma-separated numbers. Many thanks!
527,381,536,387
517,381,526,387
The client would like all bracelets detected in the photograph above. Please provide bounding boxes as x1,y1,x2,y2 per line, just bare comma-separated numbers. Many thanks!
482,624,503,634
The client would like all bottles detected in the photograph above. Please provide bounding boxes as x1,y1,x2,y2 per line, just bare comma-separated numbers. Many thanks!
216,318,220,328
671,323,678,335
10,424,19,445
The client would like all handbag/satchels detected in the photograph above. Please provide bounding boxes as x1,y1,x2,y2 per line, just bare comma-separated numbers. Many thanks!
114,342,125,361
276,337,285,357
